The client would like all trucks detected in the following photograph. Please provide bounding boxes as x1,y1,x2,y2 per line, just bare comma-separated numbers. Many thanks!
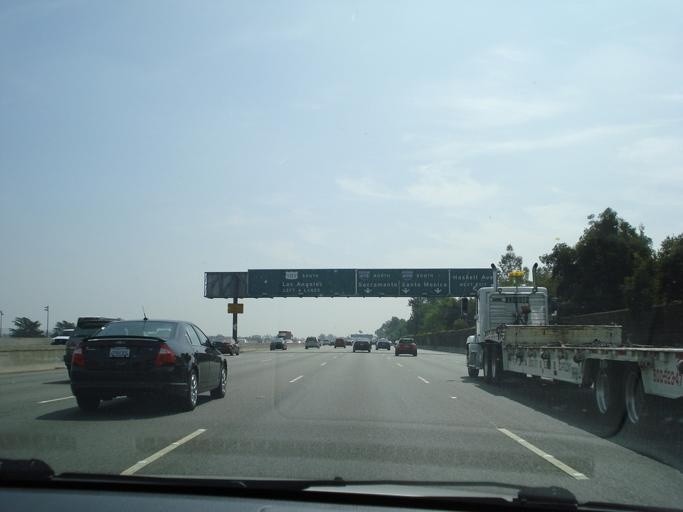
275,330,292,341
457,256,683,430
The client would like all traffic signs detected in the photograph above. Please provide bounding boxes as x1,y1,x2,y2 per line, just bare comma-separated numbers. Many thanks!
357,269,451,297
450,267,498,298
247,269,357,298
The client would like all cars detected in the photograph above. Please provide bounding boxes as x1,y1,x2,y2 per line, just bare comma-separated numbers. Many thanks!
269,336,419,356
53,315,240,412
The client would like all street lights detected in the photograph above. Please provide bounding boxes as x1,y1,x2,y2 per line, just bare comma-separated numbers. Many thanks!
0,310,4,337
44,303,50,338
